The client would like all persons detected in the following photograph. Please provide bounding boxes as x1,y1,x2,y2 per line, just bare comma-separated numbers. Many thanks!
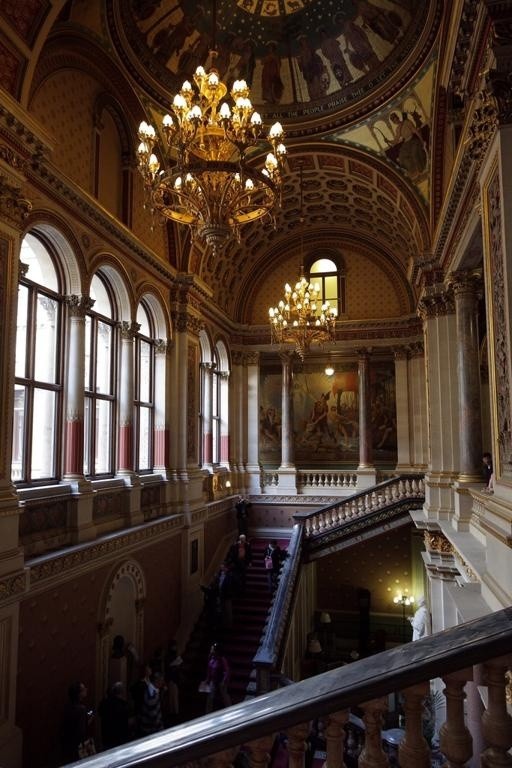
407,597,431,641
264,540,284,594
224,534,252,576
155,0,405,105
215,564,230,589
66,643,232,760
384,113,426,180
482,452,492,480
371,394,396,448
303,393,348,447
263,408,281,449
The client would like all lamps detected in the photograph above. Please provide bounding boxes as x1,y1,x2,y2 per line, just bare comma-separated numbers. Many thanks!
134,1,290,263
268,160,337,363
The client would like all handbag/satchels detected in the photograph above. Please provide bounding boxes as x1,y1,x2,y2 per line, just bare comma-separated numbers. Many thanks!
77,736,97,758
197,677,212,694
264,555,273,569
